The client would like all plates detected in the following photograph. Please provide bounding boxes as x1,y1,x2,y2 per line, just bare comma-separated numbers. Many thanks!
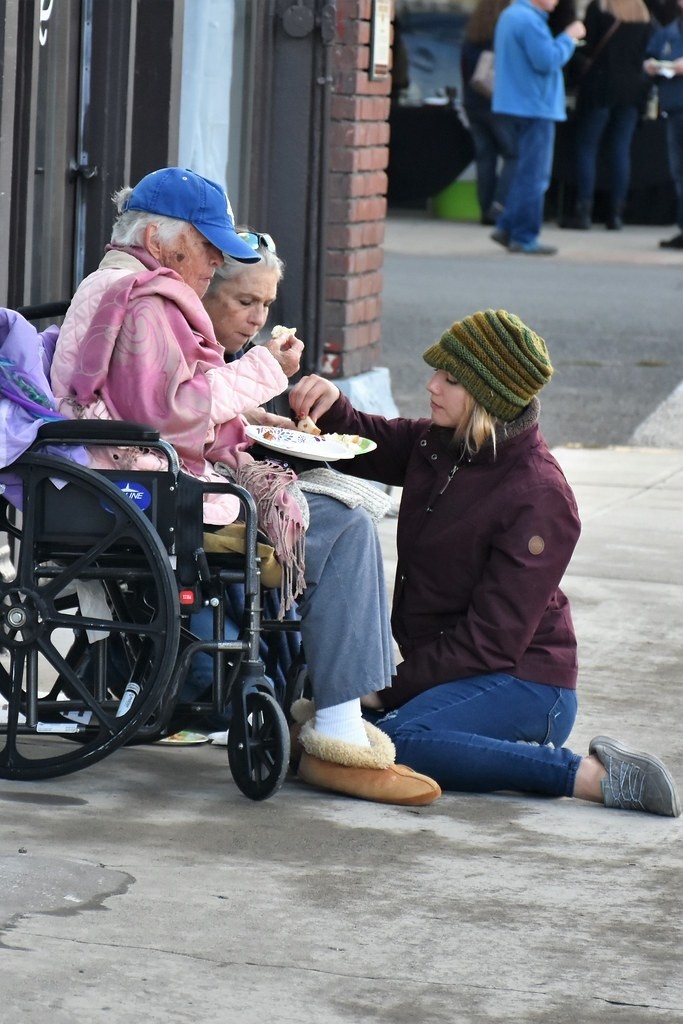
244,425,355,462
153,730,208,746
320,434,377,455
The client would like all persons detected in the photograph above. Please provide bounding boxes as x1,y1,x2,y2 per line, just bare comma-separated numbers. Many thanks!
289,309,683,818
461,0,683,250
490,0,586,255
49,166,442,805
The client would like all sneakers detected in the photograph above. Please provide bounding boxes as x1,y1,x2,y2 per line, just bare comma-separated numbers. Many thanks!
590,735,680,817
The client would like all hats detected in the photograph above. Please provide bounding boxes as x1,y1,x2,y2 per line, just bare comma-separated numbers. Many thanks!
422,309,554,422
121,167,261,264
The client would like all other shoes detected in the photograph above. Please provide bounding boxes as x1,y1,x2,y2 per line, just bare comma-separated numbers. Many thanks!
509,240,559,255
489,226,510,249
659,233,683,249
297,715,442,806
291,699,319,752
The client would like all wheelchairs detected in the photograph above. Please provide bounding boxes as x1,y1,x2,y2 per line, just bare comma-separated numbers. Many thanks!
0,420,310,801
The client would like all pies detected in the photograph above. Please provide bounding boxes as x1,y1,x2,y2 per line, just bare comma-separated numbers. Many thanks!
298,416,321,437
271,325,297,339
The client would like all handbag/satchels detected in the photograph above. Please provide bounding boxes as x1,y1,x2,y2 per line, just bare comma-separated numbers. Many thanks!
469,49,493,97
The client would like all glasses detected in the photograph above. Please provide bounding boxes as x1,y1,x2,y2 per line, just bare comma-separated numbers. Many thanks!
237,231,276,253
